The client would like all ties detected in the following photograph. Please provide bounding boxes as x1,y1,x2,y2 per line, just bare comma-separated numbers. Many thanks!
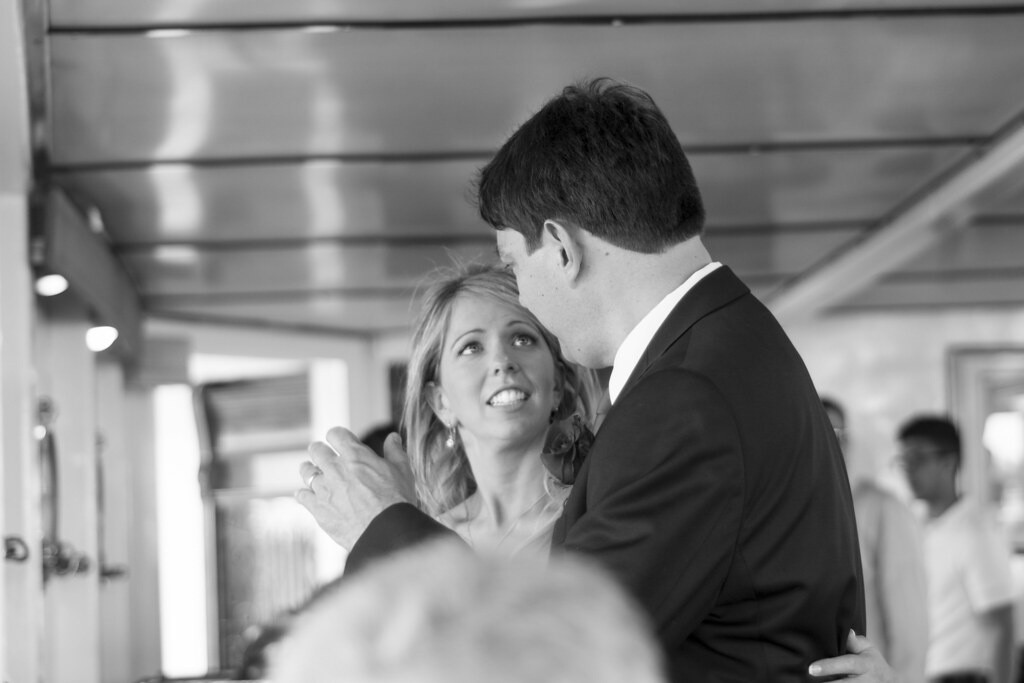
592,387,613,437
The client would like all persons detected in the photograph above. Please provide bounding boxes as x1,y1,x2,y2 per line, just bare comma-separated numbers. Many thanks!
264,536,664,683
897,414,1019,683
295,77,868,683
822,397,930,683
402,260,901,683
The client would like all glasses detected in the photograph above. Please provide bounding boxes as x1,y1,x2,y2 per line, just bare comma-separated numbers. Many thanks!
902,449,944,472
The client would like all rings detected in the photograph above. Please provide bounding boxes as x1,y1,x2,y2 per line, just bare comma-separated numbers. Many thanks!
307,471,320,489
